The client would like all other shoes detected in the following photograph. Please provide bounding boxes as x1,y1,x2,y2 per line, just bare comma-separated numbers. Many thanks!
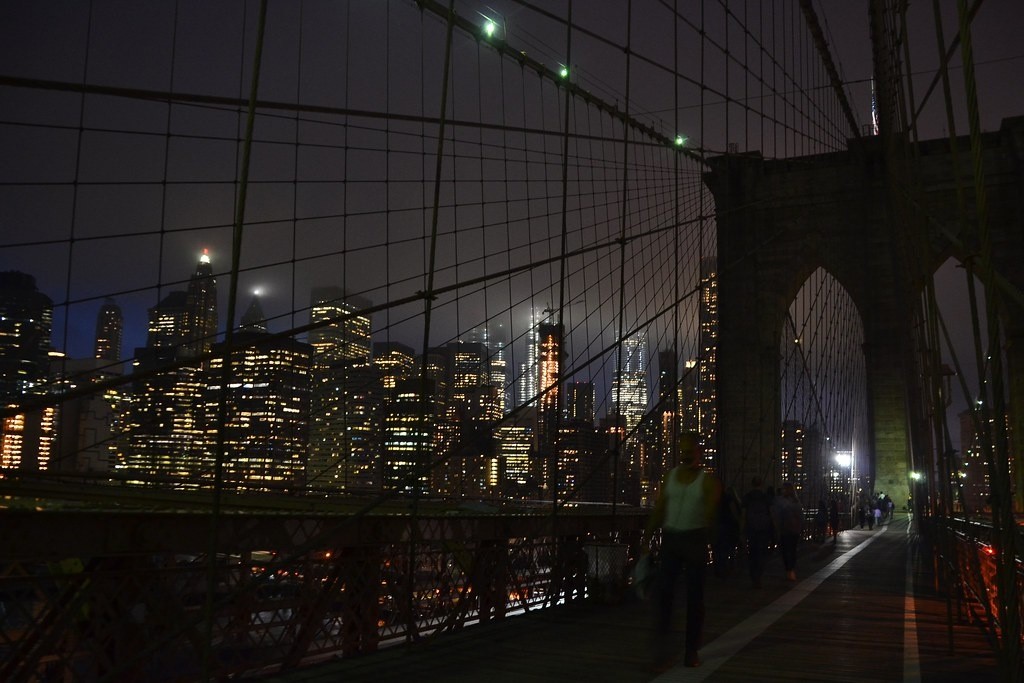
684,650,700,667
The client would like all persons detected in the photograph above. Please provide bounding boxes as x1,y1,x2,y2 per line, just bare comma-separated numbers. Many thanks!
638,432,717,673
908,500,912,511
708,475,803,581
812,500,839,545
857,492,895,530
